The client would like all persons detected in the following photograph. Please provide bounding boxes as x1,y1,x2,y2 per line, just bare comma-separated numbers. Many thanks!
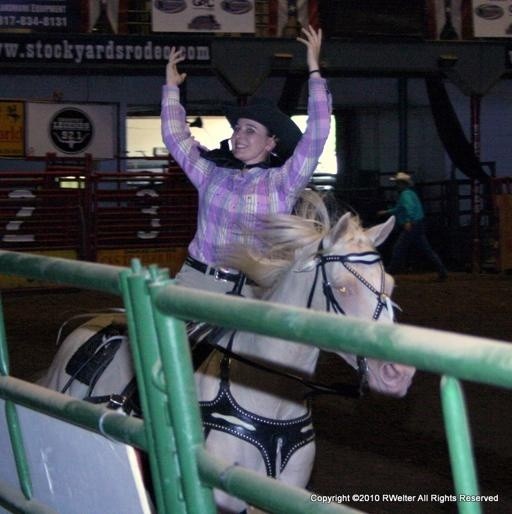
86,25,333,409
378,170,450,282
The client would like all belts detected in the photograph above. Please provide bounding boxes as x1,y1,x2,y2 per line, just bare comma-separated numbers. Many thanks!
184,254,254,286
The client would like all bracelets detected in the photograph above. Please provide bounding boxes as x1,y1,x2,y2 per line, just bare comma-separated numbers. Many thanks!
307,69,320,76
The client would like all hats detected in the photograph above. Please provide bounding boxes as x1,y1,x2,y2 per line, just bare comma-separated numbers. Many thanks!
387,171,415,188
218,95,303,159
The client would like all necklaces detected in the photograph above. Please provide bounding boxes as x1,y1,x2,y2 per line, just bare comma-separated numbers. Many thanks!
237,161,266,169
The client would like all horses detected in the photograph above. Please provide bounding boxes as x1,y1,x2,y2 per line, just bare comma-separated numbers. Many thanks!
32,188,418,514
